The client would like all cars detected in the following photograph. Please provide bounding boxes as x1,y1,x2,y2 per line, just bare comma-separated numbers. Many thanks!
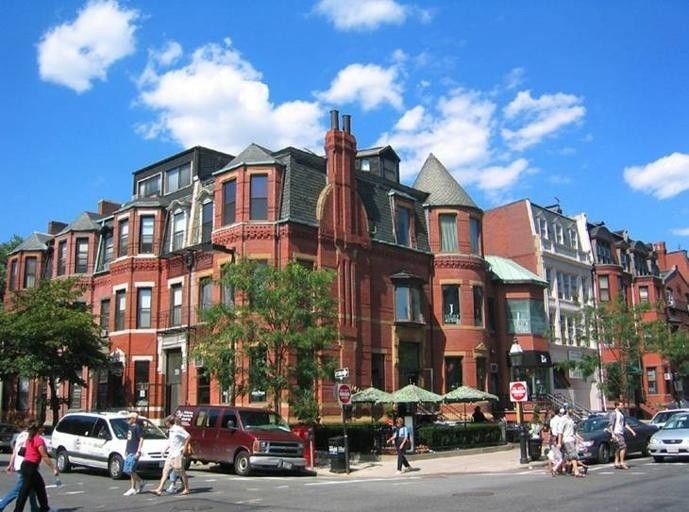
1,423,19,451
574,415,658,463
647,410,689,462
10,425,54,456
651,409,685,429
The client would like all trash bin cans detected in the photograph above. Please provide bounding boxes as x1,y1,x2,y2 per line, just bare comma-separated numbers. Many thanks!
528,439,543,461
328,436,349,473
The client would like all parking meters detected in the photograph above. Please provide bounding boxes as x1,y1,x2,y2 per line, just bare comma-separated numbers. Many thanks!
306,426,315,471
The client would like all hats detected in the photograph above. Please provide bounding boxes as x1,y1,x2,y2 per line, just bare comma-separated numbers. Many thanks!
126,412,138,418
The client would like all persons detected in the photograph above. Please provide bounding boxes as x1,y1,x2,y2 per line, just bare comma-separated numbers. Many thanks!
14,420,59,512
472,406,488,423
540,404,589,478
166,415,187,494
121,412,145,496
127,401,135,412
149,415,192,496
0,416,40,512
610,401,636,469
386,416,412,475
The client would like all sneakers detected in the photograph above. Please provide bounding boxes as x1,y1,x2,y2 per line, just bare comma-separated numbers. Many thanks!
150,490,161,495
166,487,177,493
178,488,189,494
137,480,144,493
124,489,136,495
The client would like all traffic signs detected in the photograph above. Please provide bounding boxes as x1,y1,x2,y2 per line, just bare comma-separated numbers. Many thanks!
334,368,351,379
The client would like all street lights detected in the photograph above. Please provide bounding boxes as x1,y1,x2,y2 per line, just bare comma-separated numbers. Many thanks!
508,336,532,464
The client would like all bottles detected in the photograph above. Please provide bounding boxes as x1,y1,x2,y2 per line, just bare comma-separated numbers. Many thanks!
55,475,63,488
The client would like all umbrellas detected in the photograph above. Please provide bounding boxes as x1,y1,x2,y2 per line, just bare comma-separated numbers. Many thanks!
374,379,444,415
443,384,500,427
351,385,390,425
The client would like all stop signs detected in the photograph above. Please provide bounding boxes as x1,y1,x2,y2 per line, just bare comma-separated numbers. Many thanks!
509,381,529,403
336,384,351,405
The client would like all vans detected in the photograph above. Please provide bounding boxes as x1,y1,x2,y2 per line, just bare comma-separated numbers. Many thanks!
171,403,308,476
51,411,170,479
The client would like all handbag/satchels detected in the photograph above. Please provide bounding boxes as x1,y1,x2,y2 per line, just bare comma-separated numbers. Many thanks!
184,443,195,456
18,447,26,457
